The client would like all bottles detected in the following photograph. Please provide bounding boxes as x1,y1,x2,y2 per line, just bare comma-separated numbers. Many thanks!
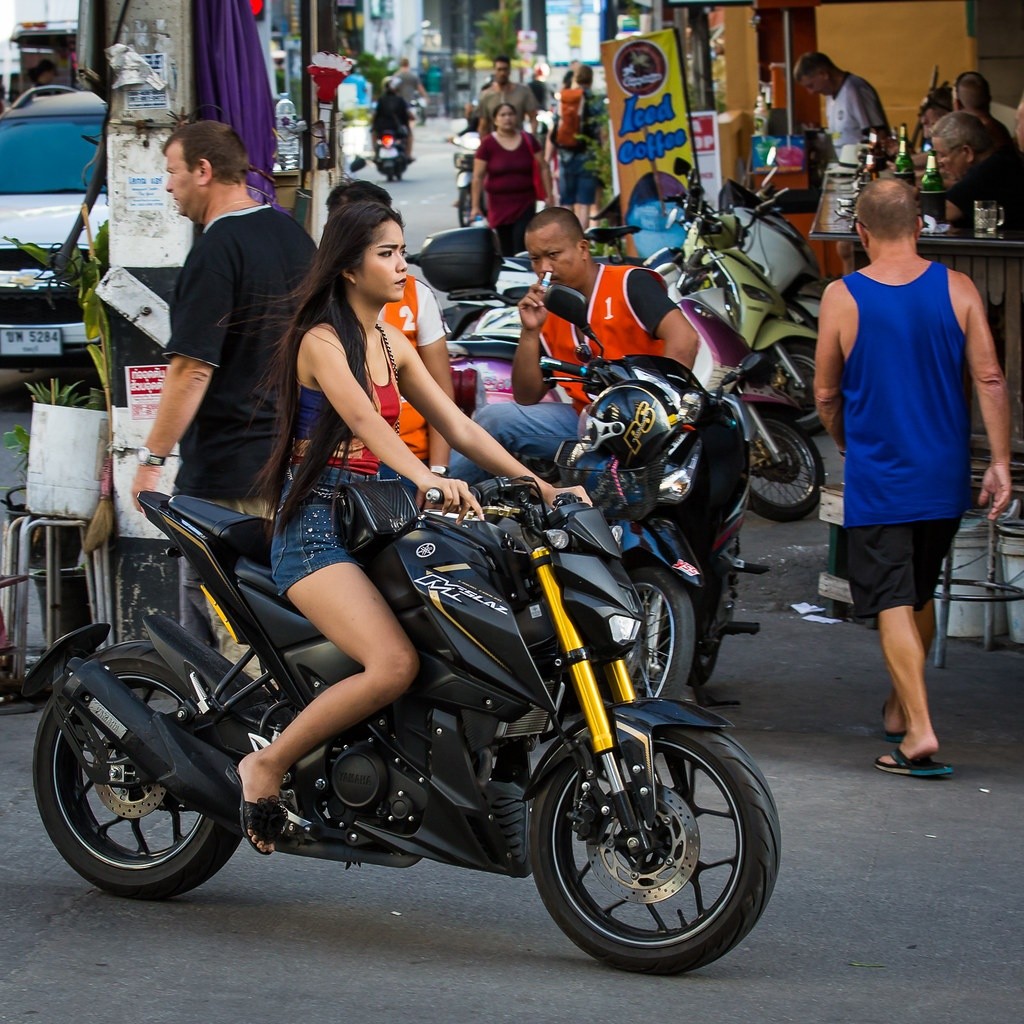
753,95,768,137
920,149,946,225
274,93,300,171
895,123,916,185
866,126,881,158
863,147,878,183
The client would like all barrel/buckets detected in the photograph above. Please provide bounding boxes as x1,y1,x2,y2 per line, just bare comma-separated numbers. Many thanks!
929,509,1024,644
30,567,91,645
5,484,82,569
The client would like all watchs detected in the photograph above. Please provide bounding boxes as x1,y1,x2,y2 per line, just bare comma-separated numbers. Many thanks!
135,446,166,466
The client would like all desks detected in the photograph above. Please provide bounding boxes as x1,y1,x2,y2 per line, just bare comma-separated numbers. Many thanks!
808,200,1024,456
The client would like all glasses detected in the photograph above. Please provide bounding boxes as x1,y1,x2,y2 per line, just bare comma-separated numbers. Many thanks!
936,143,960,163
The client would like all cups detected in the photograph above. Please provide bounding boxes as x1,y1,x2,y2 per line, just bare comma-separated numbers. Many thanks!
754,141,777,166
972,201,1005,239
775,146,804,167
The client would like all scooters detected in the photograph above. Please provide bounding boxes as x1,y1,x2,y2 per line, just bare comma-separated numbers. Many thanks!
453,131,485,227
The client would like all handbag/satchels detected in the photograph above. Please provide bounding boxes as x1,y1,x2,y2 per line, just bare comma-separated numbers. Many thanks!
334,478,420,556
523,131,548,200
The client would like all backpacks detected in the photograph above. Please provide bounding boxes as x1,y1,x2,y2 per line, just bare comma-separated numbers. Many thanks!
550,87,598,154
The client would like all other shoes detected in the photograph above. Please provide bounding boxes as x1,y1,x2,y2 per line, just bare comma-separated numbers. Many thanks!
406,157,416,165
368,156,376,162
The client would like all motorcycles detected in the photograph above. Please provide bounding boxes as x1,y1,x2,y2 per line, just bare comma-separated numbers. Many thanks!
372,130,416,183
404,144,839,699
18,473,781,977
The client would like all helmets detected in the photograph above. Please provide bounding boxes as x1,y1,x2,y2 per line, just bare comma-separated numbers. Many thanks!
382,76,402,93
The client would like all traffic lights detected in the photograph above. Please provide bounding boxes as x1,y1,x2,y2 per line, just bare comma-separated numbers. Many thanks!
250,0,266,20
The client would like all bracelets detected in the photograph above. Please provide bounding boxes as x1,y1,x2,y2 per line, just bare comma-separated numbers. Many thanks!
430,464,449,476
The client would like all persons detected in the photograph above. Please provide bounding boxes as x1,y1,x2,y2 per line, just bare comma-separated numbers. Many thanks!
324,176,454,517
368,77,413,182
454,57,609,224
470,103,555,229
274,57,287,97
922,72,1024,222
813,179,1011,777
27,59,62,101
236,201,594,855
793,51,892,172
391,58,428,132
131,120,331,692
443,207,699,583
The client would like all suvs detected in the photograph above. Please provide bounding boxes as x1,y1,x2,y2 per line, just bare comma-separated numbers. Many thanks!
0,86,110,373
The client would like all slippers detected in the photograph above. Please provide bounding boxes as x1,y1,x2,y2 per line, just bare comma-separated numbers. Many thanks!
874,748,952,776
881,699,907,742
226,765,285,855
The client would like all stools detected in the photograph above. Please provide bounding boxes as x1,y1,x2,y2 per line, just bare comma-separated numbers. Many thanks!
933,457,1024,670
